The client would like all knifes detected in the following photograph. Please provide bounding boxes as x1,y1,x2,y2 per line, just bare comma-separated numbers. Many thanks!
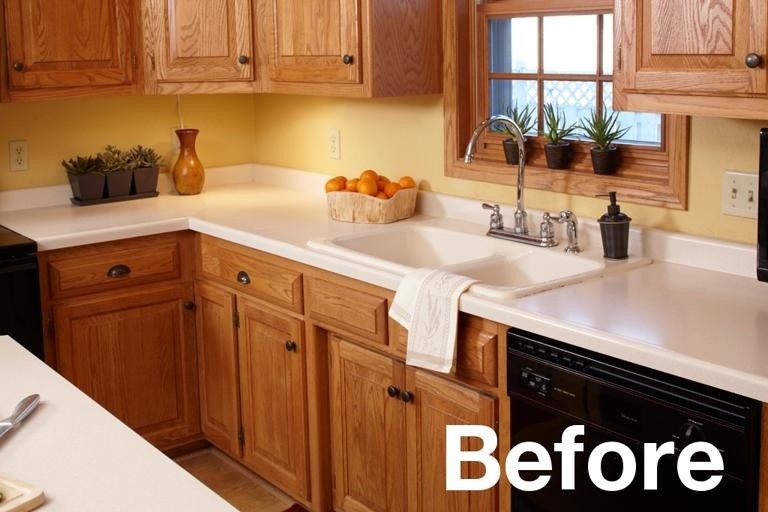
0,392,41,446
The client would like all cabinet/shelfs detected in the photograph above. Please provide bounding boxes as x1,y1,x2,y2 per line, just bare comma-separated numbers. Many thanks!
611,1,768,122
264,0,441,101
0,1,139,104
307,262,503,512
196,229,310,512
38,231,200,457
141,1,264,97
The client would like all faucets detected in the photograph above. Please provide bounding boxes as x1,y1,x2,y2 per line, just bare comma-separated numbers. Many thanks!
464,113,528,235
544,210,579,254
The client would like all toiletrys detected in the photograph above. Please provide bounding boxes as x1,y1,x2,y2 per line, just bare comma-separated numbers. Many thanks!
595,191,629,261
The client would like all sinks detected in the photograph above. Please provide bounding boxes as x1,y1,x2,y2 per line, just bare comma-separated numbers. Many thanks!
443,250,602,290
332,222,510,271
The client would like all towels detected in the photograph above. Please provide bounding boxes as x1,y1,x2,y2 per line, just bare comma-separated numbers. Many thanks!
388,255,510,376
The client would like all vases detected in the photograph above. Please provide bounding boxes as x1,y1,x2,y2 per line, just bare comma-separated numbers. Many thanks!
172,128,205,195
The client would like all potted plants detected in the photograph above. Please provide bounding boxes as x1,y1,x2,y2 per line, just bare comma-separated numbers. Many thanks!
122,145,169,194
94,144,133,197
493,98,537,166
60,153,106,200
576,104,633,176
533,102,578,170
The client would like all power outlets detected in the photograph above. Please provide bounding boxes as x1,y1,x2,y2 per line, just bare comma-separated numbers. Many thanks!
9,139,27,171
